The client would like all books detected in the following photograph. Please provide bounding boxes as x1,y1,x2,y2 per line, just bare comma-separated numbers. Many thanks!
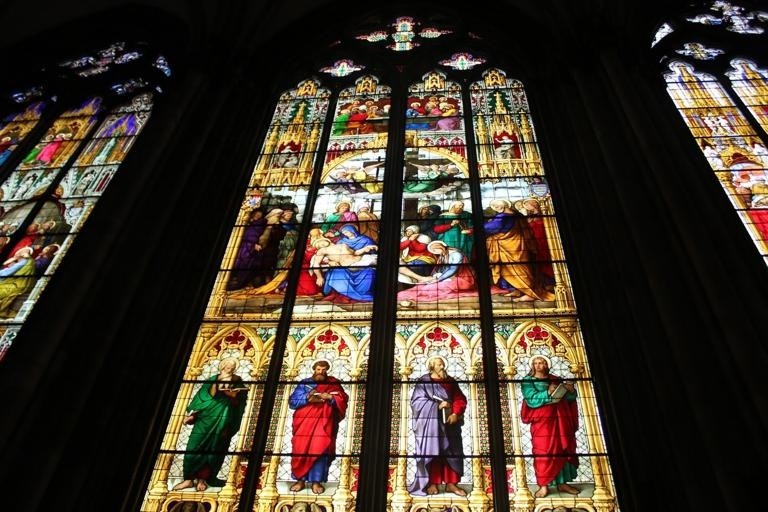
442,399,463,425
307,393,325,405
217,387,249,394
546,383,569,404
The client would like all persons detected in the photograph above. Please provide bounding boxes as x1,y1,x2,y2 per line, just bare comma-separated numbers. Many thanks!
519,352,581,500
675,59,768,212
286,361,349,497
170,356,251,494
0,111,146,318
407,356,471,499
224,94,556,308
